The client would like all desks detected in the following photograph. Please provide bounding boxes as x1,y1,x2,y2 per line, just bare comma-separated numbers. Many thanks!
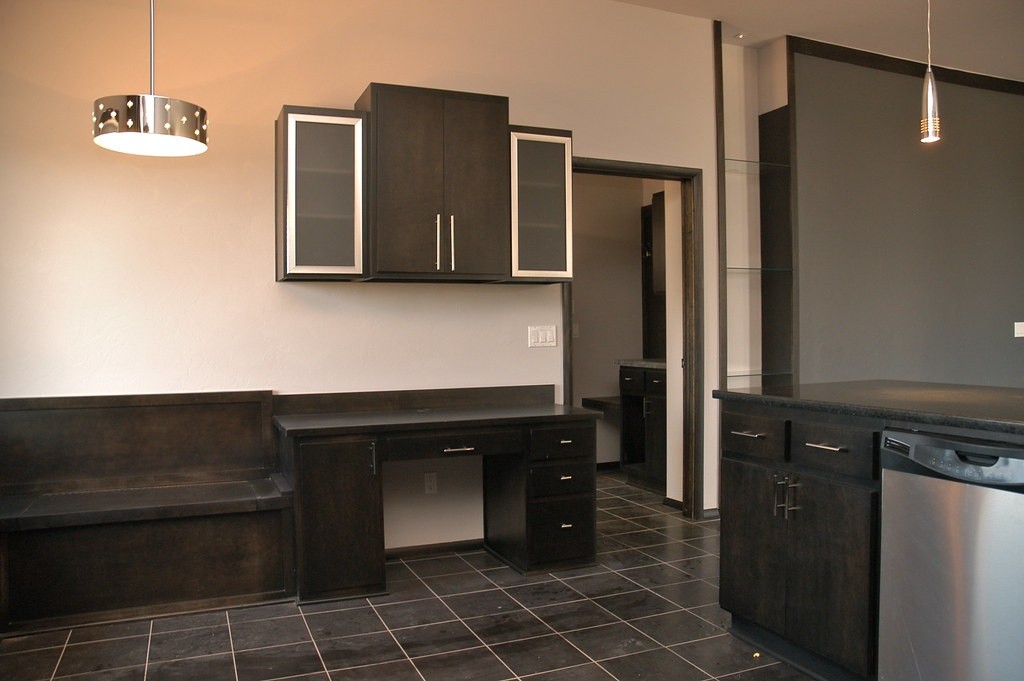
273,403,604,608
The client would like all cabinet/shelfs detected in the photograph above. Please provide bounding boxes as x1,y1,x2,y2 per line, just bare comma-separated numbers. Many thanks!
513,126,580,280
724,156,794,379
275,104,364,280
353,82,517,281
619,368,666,483
718,413,881,679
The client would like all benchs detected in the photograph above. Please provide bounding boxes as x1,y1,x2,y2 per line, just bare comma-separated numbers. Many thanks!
0,391,295,637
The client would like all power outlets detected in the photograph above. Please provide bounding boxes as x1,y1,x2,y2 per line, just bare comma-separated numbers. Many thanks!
420,472,437,493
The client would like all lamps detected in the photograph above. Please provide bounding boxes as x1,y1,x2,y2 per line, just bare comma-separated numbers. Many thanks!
91,1,209,159
919,1,942,144
